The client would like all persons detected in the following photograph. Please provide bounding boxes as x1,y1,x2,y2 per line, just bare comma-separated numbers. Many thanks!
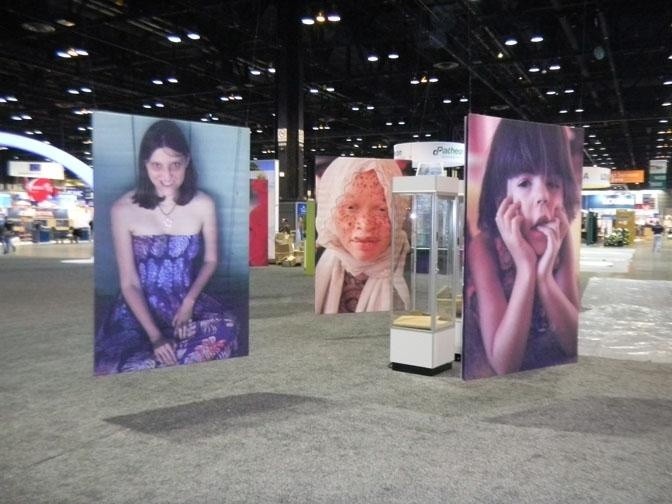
468,118,578,375
651,220,665,253
0,213,94,255
279,217,290,234
95,111,249,376
315,157,411,313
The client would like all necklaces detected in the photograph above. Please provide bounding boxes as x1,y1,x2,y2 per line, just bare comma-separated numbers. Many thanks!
155,190,180,228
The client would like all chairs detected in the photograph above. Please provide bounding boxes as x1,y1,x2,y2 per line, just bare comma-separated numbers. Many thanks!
10,213,75,246
